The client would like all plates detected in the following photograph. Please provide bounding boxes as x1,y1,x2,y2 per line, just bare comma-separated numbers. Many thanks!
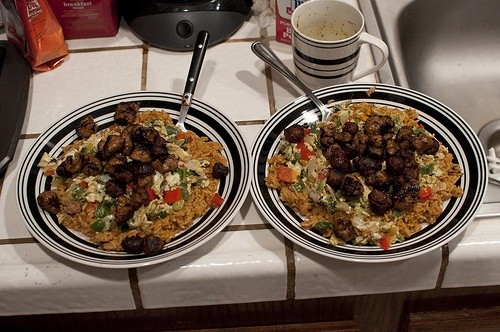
15,90,252,268
250,82,489,264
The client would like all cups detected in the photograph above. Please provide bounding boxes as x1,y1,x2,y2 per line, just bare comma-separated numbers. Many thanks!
290,0,389,92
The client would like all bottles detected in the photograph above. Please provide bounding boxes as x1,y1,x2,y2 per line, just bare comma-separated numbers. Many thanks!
269,1,311,54
